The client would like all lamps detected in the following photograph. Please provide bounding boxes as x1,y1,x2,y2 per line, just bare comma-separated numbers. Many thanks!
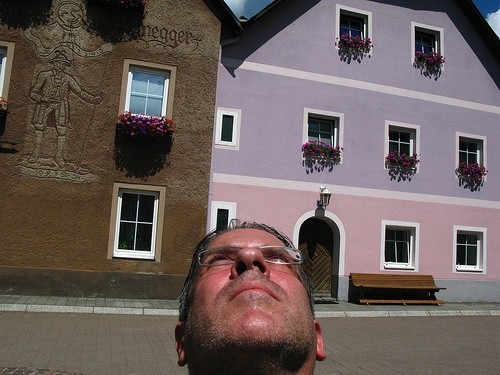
317,184,332,209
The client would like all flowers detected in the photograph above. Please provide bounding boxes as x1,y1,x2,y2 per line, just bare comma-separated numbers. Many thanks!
335,33,373,58
386,150,420,168
455,162,489,182
116,112,177,142
414,49,446,73
302,140,344,164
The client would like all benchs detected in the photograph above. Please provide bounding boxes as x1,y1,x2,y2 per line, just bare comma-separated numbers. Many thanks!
349,273,447,305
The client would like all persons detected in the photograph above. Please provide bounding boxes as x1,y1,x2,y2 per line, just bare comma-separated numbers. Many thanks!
173,219,326,375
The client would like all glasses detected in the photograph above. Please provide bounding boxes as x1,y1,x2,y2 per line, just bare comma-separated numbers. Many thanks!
188,245,305,279
